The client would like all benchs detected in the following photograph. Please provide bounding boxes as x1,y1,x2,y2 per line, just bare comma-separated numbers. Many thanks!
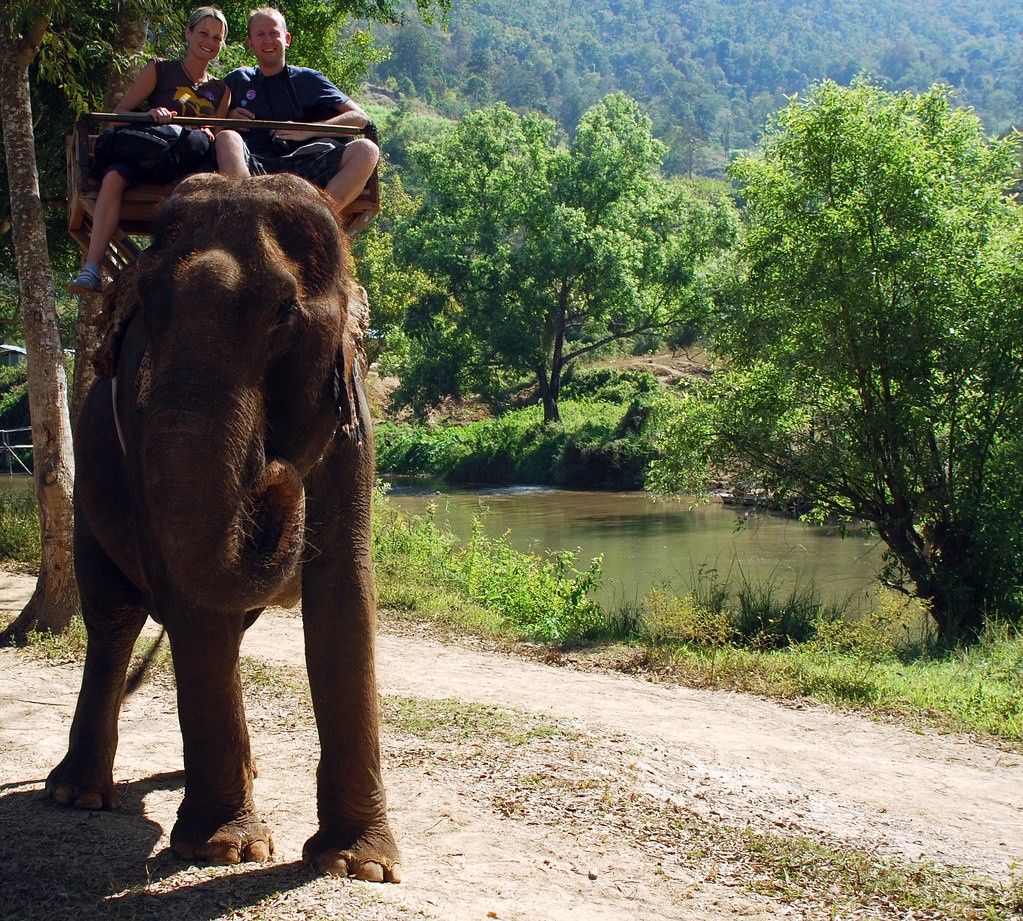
66,112,380,280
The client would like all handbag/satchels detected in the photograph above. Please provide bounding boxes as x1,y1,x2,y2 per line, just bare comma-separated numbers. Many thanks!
93,120,220,189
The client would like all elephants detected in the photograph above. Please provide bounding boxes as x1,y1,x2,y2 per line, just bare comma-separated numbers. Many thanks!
42,170,405,887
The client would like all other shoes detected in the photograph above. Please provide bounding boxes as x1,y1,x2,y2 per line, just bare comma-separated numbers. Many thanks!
68,271,104,295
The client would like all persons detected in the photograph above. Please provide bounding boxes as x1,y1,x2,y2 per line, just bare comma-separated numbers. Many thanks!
69,7,231,294
215,7,379,215
